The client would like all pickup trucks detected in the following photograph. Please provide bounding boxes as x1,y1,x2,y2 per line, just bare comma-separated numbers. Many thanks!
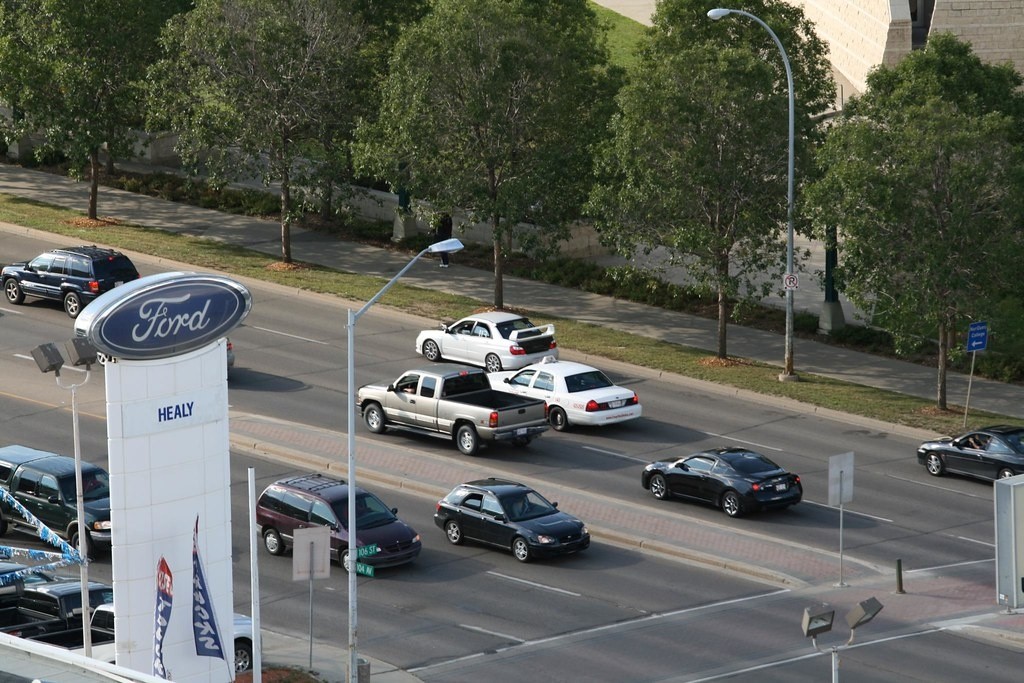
0,562,81,607
24,602,263,675
0,579,113,639
0,444,111,560
356,362,549,455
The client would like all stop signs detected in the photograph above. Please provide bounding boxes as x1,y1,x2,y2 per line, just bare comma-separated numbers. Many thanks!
784,274,799,291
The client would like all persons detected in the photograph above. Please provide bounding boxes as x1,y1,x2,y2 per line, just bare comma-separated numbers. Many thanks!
512,497,530,517
968,434,990,450
436,211,452,268
403,375,420,394
83,473,102,494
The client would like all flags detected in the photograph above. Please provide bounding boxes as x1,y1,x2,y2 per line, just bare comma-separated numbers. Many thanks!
152,552,174,681
192,512,227,661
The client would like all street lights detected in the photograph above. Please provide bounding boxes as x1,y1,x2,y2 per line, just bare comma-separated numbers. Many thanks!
30,336,100,656
708,6,796,382
345,238,466,683
801,597,884,683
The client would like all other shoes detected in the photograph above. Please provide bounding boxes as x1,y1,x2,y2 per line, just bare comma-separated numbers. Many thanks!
439,264,449,268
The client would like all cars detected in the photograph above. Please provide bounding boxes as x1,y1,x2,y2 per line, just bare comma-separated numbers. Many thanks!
641,448,803,516
414,311,559,372
256,473,422,573
487,355,642,431
916,425,1024,480
434,477,591,562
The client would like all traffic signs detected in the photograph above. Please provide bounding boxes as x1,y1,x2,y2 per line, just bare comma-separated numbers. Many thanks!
967,322,987,351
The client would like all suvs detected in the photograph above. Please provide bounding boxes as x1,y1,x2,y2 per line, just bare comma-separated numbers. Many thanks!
0,244,140,319
